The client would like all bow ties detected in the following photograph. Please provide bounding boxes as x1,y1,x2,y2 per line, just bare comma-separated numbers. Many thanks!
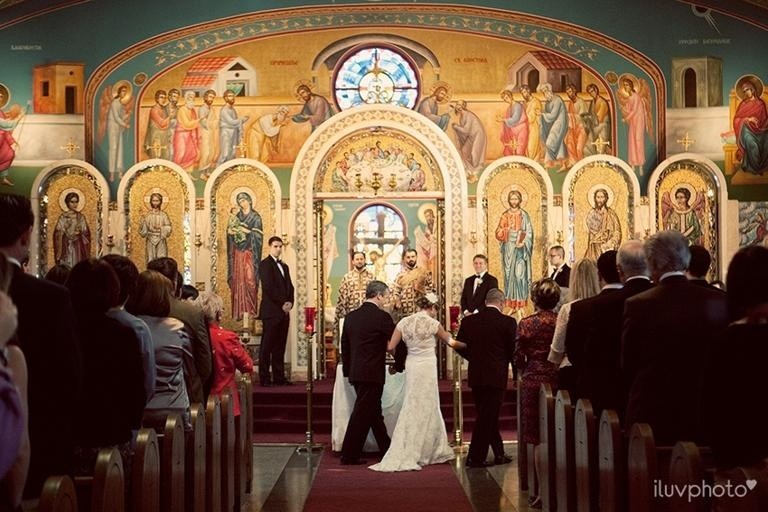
474,274,481,279
277,259,282,263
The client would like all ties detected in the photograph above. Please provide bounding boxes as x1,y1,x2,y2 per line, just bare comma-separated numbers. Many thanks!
551,268,558,279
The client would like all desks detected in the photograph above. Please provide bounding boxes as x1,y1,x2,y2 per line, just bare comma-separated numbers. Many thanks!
332,363,406,457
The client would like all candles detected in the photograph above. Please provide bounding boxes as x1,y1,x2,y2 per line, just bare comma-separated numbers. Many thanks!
303,307,316,333
448,305,458,331
243,312,249,328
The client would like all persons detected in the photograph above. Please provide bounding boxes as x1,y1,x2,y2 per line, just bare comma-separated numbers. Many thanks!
0,192,254,511
454,288,518,468
257,237,296,387
333,251,394,352
367,293,467,471
0,77,768,320
342,281,407,464
391,249,435,326
514,228,768,511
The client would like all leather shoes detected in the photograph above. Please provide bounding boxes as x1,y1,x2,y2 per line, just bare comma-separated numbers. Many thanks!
341,456,367,464
466,459,488,467
495,455,513,464
